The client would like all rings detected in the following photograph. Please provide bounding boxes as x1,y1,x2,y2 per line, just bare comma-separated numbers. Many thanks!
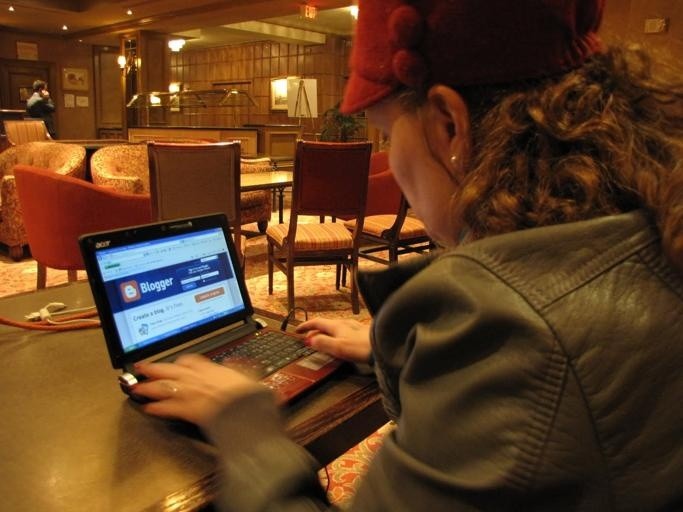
169,380,178,396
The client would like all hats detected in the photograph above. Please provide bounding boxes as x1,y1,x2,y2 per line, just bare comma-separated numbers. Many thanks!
341,0,603,114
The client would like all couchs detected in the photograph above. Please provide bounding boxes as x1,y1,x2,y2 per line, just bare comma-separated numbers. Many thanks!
89,142,272,236
331,150,405,224
0,139,87,261
10,162,151,290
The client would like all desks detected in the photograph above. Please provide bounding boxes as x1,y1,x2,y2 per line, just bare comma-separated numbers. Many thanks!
0,276,393,512
237,169,294,224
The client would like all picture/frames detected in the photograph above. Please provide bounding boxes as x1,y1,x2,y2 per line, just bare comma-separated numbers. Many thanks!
58,63,91,93
267,74,303,113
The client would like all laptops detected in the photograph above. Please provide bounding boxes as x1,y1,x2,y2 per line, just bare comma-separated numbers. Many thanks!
78,213,344,426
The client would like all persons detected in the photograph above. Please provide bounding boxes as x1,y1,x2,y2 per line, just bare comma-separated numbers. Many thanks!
25,79,57,136
124,1,683,511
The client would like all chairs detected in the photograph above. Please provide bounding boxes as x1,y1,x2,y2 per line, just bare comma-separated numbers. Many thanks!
1,118,54,148
145,138,244,279
263,136,374,315
340,193,440,288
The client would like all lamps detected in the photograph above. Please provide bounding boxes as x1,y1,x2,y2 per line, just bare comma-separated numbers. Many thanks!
117,54,130,73
